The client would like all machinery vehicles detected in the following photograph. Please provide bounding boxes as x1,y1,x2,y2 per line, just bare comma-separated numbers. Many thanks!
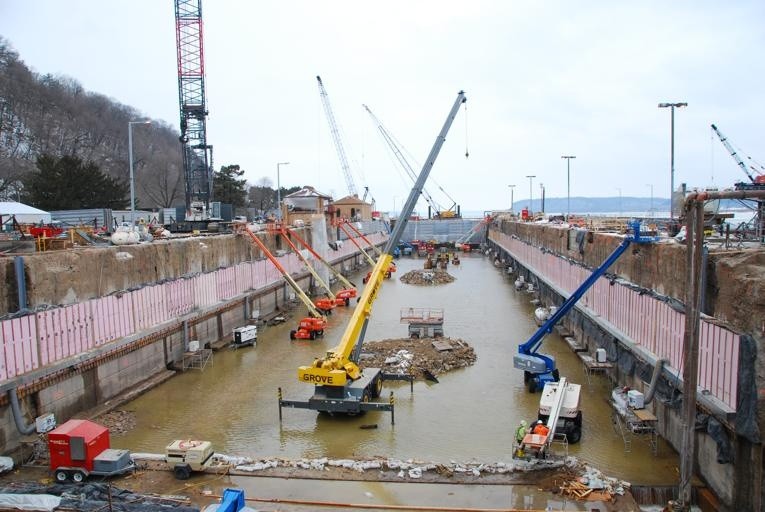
451,213,497,253
274,87,470,426
356,101,465,220
708,124,765,192
510,376,586,465
150,0,233,233
229,219,433,340
511,217,661,395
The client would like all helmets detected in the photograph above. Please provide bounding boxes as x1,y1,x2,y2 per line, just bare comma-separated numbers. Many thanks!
519,419,543,427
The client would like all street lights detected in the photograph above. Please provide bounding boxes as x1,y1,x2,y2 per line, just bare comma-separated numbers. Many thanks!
508,185,517,218
657,101,688,230
558,155,577,230
275,161,289,224
126,119,155,240
525,175,536,221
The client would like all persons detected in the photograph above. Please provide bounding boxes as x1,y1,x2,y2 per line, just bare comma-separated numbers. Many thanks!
348,349,359,365
514,415,549,460
29,223,64,250
92,225,108,239
